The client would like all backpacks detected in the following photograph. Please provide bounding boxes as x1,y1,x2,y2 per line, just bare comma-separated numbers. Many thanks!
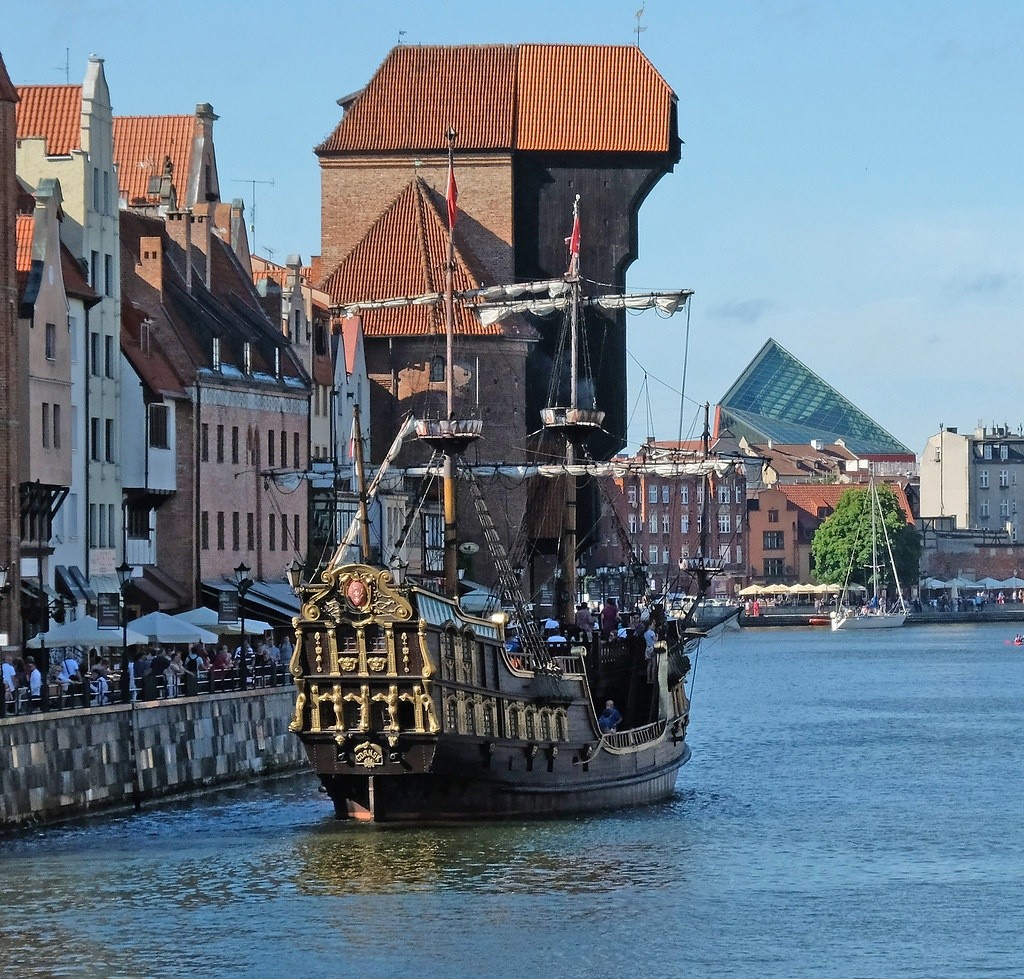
187,655,198,672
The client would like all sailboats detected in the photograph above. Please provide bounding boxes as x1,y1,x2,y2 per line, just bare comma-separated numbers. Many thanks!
829,467,913,631
286,126,743,826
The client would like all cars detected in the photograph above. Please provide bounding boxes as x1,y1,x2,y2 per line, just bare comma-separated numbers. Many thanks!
655,591,740,613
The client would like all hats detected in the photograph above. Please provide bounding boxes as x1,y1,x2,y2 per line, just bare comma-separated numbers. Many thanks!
27,656,34,663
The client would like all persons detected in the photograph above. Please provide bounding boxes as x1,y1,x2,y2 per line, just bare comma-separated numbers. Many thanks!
0,636,296,718
575,597,659,661
599,700,623,735
879,597,887,615
726,596,799,616
814,598,825,615
977,590,1024,604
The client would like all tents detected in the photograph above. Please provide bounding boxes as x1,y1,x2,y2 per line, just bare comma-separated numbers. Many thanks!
27,606,274,648
738,582,868,606
920,577,1024,598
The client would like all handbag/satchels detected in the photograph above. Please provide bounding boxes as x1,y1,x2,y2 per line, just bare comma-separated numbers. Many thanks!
69,674,79,682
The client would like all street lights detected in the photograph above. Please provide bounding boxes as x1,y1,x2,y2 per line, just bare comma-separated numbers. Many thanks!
575,560,588,605
114,560,134,705
617,561,628,612
598,562,609,609
232,560,252,691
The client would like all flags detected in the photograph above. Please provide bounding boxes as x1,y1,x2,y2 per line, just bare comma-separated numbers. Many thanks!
570,218,580,257
348,419,356,466
448,167,460,233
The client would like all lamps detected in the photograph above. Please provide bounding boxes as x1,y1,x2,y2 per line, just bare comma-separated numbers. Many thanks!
286,557,304,594
388,555,409,593
